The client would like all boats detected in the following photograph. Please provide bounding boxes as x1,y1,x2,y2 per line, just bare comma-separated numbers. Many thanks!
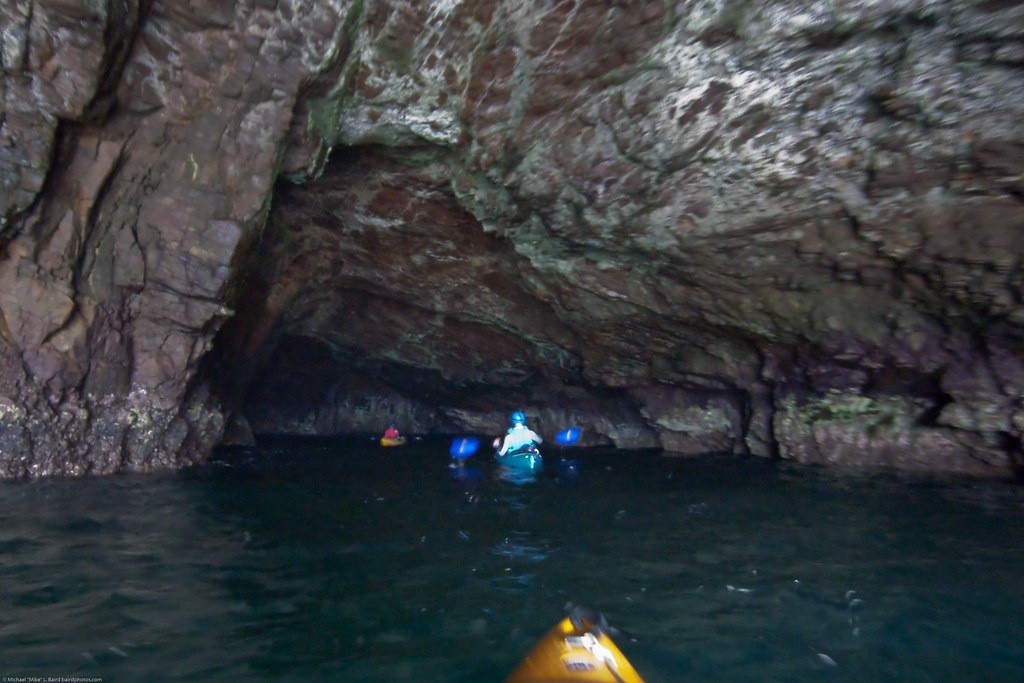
380,436,406,447
494,443,544,472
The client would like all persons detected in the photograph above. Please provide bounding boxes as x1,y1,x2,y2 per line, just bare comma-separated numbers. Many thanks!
499,411,543,456
384,423,399,439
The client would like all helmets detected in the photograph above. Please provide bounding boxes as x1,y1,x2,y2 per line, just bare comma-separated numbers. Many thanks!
512,412,526,424
